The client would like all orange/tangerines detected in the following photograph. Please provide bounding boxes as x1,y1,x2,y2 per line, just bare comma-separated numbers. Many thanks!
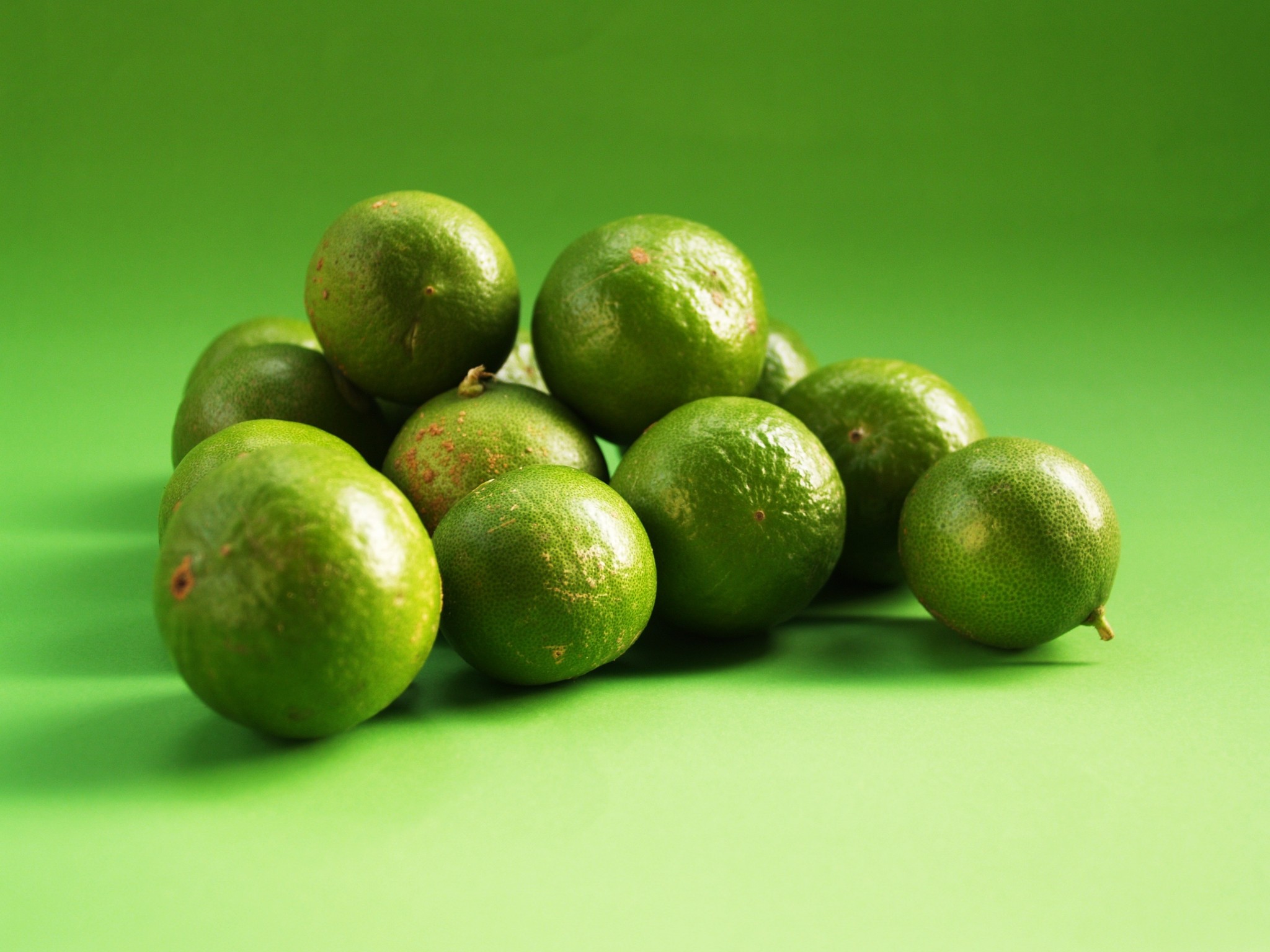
153,189,1118,745
